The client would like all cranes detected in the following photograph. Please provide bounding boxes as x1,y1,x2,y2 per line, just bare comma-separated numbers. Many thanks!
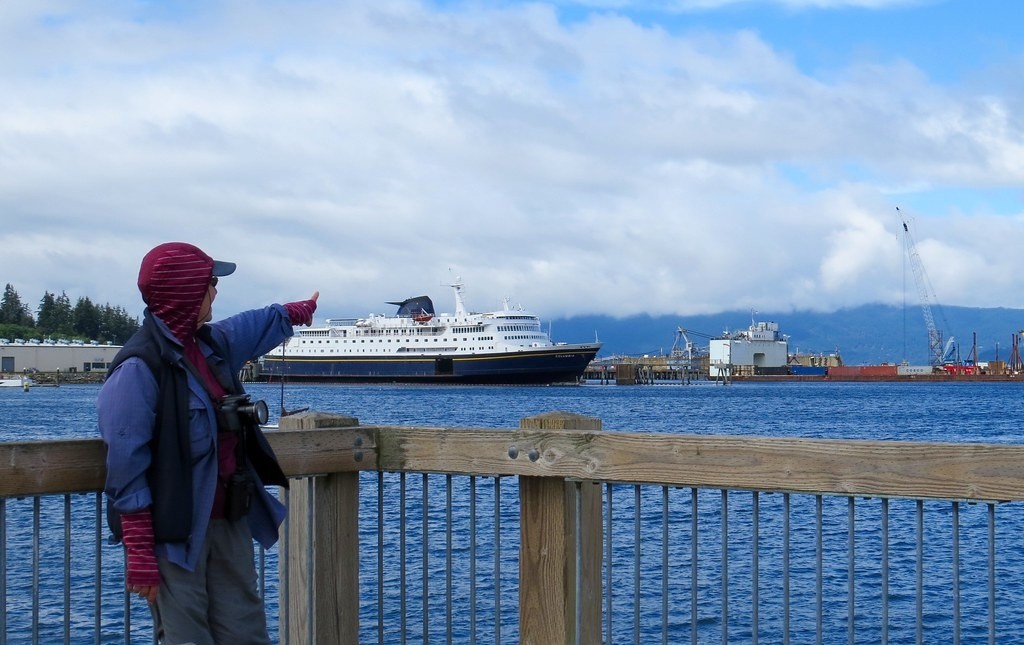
896,206,981,375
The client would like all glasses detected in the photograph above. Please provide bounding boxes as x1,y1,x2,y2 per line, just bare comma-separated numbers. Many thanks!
210,276,218,287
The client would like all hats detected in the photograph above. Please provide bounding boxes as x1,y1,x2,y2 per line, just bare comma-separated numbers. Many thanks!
210,261,236,276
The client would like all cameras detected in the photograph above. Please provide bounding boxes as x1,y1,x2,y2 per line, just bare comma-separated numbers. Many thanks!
221,394,269,436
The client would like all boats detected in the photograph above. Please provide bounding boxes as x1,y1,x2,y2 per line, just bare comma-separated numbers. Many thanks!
238,283,604,386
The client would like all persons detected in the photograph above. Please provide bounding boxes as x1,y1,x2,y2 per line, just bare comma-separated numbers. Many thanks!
98,241,319,644
